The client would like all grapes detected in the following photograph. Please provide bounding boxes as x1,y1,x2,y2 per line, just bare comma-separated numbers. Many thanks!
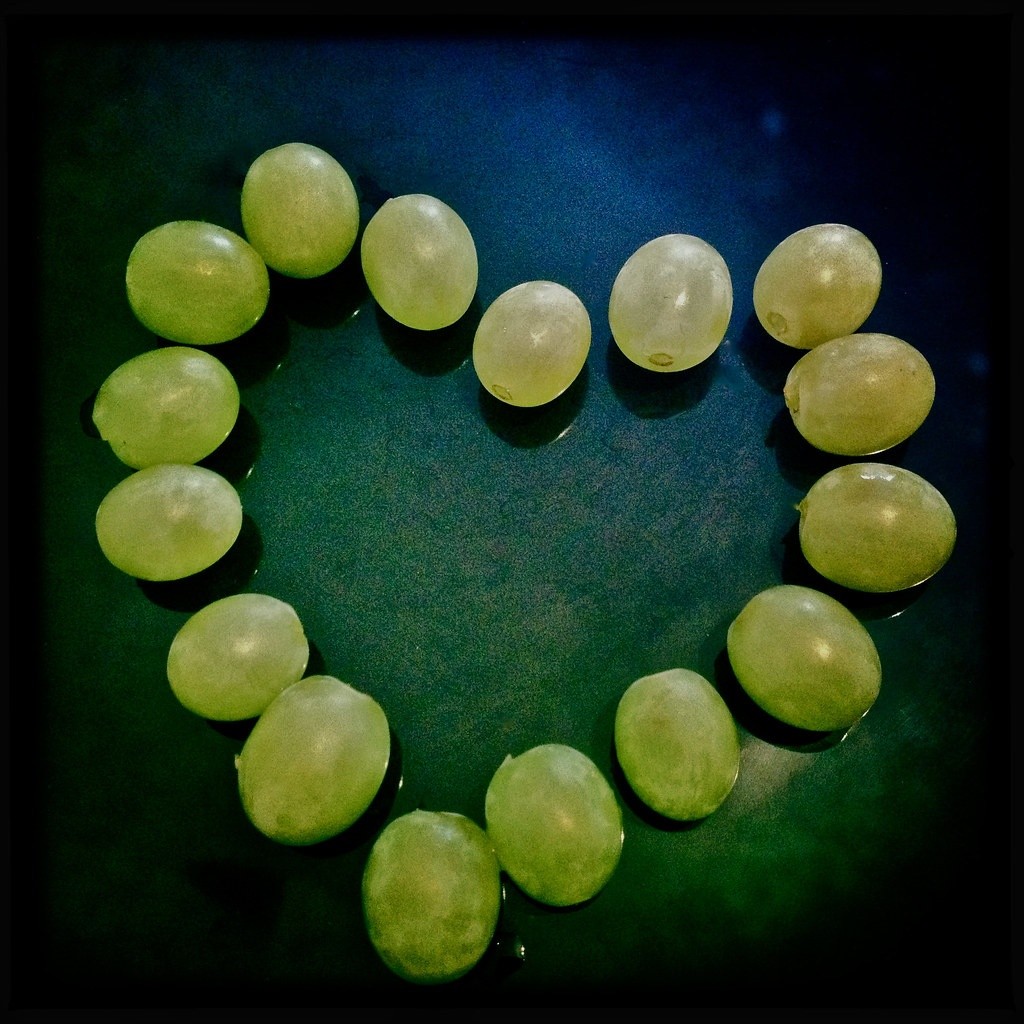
90,142,959,985
